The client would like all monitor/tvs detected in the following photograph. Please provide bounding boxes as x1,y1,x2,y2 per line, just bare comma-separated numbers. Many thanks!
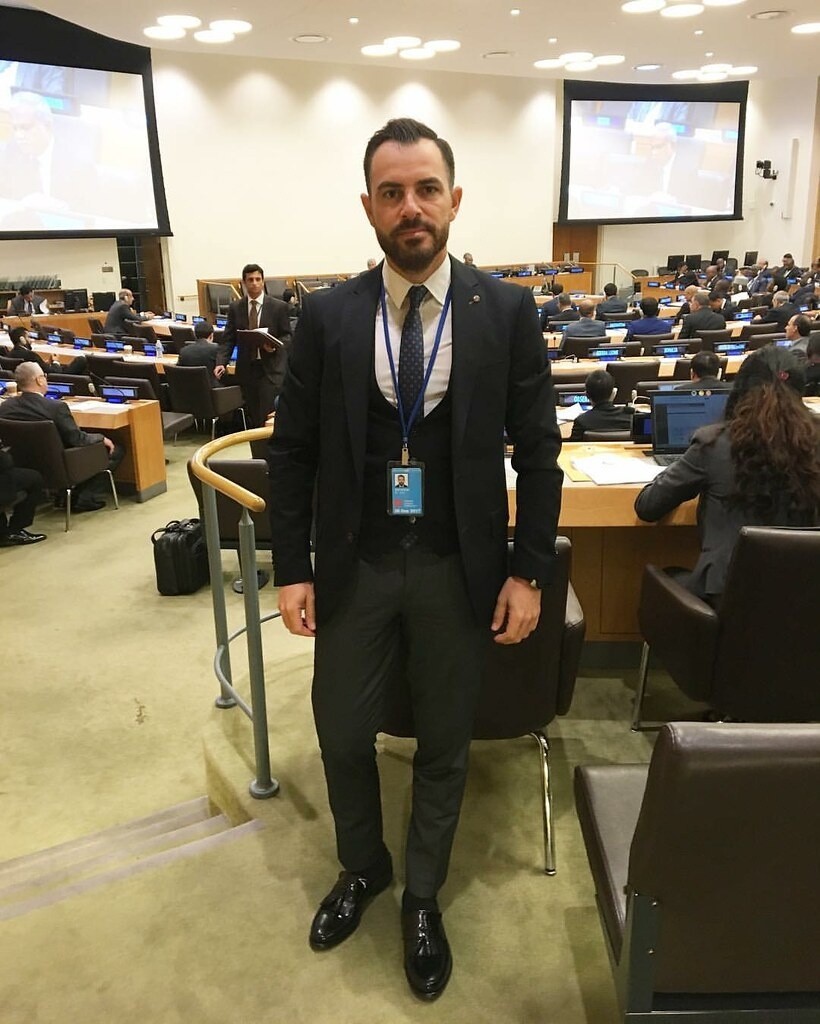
667,255,685,272
92,292,115,312
745,251,758,266
710,250,730,266
64,288,88,313
686,255,701,270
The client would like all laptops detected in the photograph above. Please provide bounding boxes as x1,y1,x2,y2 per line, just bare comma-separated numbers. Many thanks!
648,390,732,466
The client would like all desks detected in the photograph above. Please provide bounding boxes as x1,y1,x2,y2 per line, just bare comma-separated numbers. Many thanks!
142,314,218,336
548,355,748,378
0,391,167,504
502,439,699,643
0,329,237,374
533,293,606,309
0,311,108,339
627,306,682,317
542,320,751,348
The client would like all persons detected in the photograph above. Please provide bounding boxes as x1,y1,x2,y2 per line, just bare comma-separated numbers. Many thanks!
177,321,234,437
8,328,87,373
541,254,820,444
464,253,476,268
9,286,50,316
634,346,820,604
0,450,47,545
368,259,376,269
0,362,123,509
266,117,564,1001
395,475,407,487
104,289,155,339
284,288,300,310
215,264,291,429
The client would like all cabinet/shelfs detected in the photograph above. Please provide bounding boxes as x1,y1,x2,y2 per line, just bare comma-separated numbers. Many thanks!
117,235,148,313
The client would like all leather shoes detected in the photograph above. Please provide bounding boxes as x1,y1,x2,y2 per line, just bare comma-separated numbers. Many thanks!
53,495,68,510
400,890,452,997
310,854,395,948
0,523,47,546
71,495,106,511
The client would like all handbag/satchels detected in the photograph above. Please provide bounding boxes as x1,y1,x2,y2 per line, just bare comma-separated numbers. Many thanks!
151,515,208,596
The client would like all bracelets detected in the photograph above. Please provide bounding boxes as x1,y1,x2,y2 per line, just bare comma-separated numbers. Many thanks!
530,578,544,588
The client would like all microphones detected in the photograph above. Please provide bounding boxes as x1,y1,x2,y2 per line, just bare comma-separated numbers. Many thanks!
86,370,130,404
16,313,26,328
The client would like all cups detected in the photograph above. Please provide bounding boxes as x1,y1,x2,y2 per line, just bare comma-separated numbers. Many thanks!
124,345,132,357
6,381,17,396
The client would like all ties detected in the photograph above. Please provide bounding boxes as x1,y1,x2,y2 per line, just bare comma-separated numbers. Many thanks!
28,300,32,314
249,300,258,361
394,283,429,550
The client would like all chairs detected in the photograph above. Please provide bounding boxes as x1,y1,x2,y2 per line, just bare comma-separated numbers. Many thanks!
0,258,820,1024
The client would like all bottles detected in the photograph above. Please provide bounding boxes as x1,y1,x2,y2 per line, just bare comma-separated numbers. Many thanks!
155,339,163,359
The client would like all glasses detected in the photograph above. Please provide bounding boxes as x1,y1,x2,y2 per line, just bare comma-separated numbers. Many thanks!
31,373,48,382
